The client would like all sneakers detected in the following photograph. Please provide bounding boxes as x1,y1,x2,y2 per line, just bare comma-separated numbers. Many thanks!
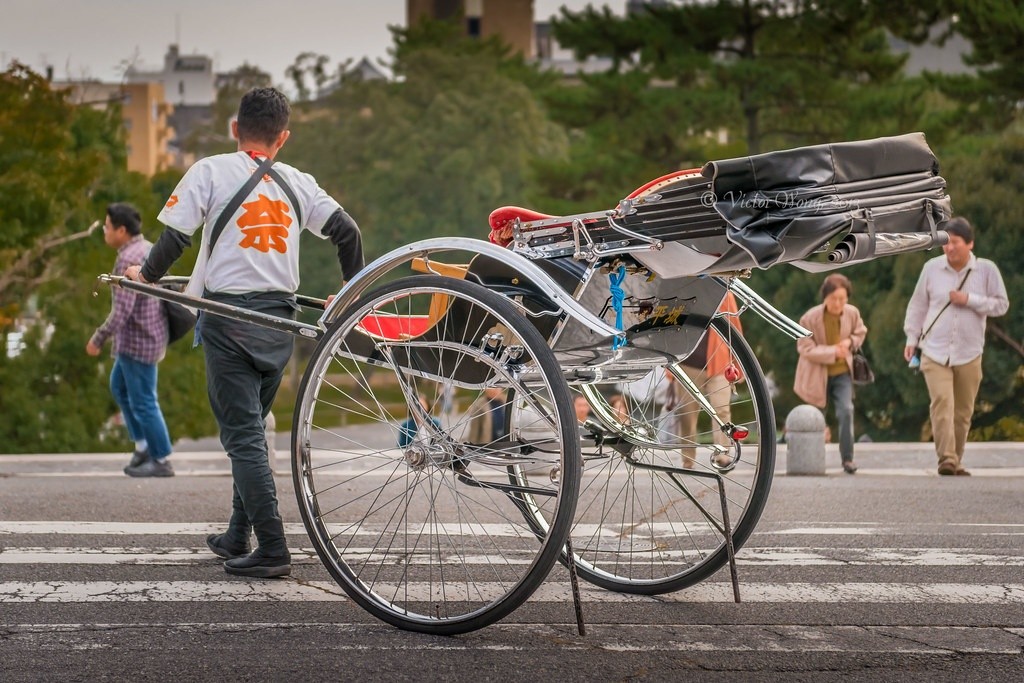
128,459,174,477
223,548,291,577
206,533,252,559
124,446,149,474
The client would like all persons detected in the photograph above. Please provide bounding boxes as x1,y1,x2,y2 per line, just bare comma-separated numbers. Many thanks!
920,402,934,442
468,387,506,446
397,394,440,446
611,396,648,439
904,218,1009,475
825,425,831,443
664,291,744,475
574,394,590,439
123,88,364,575
794,275,866,473
87,204,175,477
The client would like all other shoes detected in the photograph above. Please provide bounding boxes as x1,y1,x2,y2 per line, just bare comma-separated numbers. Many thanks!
956,469,971,476
842,459,857,472
710,452,735,470
938,463,956,475
683,461,695,469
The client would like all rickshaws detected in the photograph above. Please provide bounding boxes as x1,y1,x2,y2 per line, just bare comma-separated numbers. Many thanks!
97,131,955,637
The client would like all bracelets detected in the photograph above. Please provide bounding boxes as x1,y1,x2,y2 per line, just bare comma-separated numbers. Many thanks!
137,272,141,283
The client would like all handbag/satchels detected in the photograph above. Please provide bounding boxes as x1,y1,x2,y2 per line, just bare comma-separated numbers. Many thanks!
162,274,198,345
913,347,922,375
853,344,874,385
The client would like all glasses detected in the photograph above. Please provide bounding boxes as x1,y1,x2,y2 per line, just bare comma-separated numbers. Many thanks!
103,225,109,234
